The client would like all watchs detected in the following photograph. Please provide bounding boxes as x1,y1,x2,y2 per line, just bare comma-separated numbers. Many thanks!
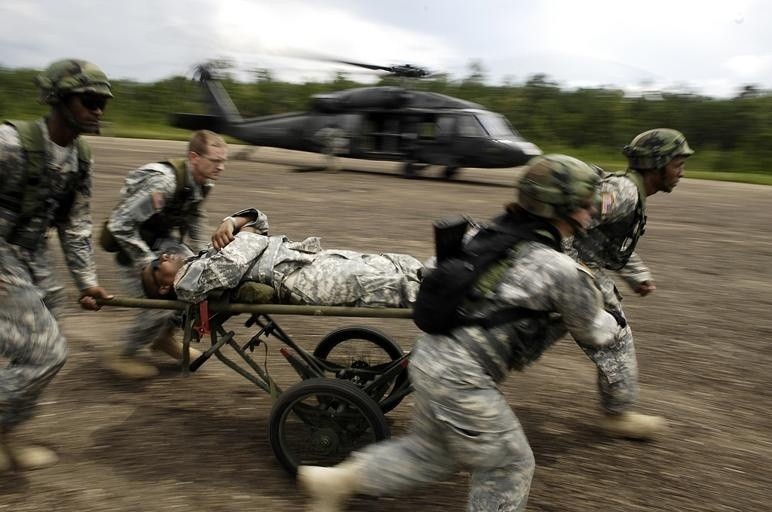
222,216,238,231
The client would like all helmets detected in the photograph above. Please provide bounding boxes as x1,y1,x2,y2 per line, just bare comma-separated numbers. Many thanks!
516,153,604,219
33,59,114,104
623,128,695,169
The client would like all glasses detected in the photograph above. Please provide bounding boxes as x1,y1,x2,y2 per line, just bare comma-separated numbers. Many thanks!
75,95,105,110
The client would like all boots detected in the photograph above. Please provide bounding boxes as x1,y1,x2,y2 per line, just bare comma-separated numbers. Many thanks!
152,327,203,362
101,347,160,379
600,411,667,438
296,461,361,512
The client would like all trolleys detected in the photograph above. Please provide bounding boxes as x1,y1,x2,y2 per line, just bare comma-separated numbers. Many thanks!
78,295,418,480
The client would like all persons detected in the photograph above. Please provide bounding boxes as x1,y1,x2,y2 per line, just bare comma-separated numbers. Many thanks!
546,127,695,440
0,59,113,473
314,123,349,174
295,153,618,512
98,128,227,381
141,206,424,308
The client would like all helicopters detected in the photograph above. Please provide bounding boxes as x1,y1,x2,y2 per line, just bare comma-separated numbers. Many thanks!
164,50,543,180
